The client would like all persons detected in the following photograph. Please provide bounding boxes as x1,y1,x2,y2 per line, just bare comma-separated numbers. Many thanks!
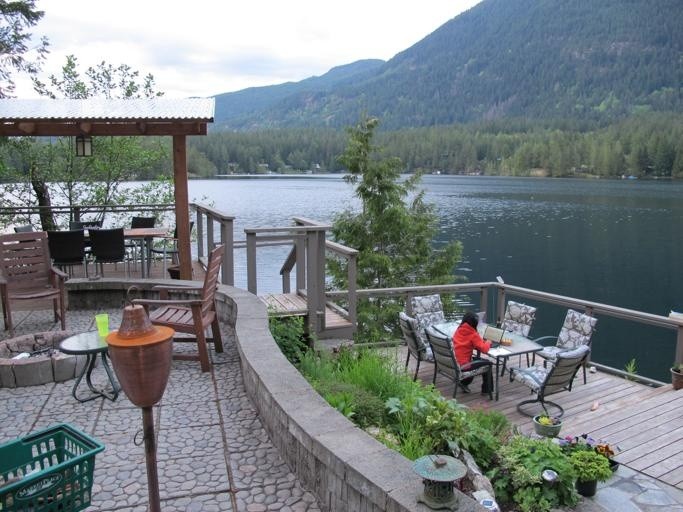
452,312,496,396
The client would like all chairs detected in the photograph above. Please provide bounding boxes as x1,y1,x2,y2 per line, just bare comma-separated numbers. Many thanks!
397,293,598,419
0,215,226,374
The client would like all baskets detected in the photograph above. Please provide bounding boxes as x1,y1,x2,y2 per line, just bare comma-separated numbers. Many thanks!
0,422,104,511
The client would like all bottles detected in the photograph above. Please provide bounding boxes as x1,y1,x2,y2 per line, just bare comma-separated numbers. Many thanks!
509,329,513,341
496,316,501,328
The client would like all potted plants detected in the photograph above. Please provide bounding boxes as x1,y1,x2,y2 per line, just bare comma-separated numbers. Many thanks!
669,362,683,390
565,450,614,498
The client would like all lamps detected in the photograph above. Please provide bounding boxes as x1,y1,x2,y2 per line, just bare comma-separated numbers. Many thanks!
75,131,93,158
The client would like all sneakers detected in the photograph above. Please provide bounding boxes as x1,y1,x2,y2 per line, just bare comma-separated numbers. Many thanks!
460,382,471,393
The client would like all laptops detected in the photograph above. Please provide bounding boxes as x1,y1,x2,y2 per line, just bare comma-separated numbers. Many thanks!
482,324,505,348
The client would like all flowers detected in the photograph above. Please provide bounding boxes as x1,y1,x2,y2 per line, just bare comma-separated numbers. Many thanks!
532,412,622,472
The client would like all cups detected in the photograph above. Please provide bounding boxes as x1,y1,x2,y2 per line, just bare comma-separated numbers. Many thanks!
95,313,109,338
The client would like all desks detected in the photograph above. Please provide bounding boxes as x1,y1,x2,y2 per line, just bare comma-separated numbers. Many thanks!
57,328,122,403
